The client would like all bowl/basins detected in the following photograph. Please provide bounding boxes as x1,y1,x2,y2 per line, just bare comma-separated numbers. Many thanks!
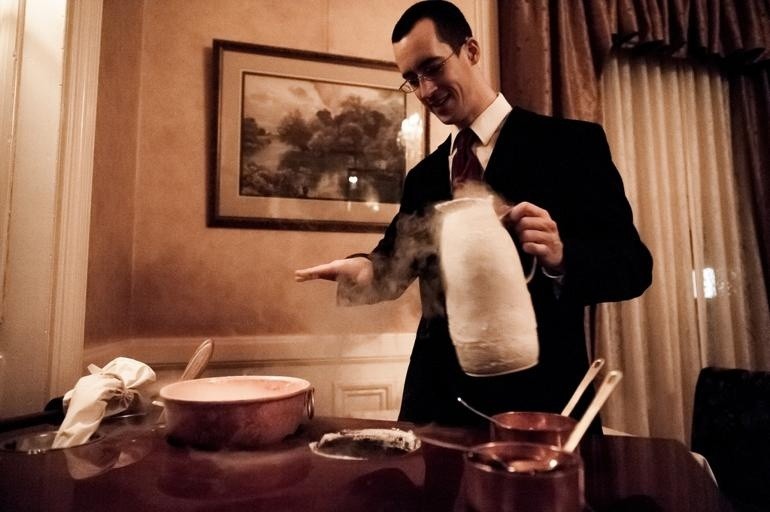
0,429,106,467
489,411,580,448
462,442,585,512
159,375,316,449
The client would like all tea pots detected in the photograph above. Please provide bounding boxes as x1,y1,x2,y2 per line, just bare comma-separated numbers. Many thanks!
433,192,540,378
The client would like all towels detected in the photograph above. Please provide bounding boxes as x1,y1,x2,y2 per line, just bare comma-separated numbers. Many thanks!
50,355,156,446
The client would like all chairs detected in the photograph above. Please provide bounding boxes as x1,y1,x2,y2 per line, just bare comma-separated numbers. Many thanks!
693,368,770,511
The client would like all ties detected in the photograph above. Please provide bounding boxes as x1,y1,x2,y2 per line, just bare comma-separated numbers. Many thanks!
451,130,484,188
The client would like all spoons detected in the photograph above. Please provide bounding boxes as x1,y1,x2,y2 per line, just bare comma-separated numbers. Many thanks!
417,433,558,474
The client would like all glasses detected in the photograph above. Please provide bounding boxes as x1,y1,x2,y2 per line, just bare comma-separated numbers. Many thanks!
399,40,468,94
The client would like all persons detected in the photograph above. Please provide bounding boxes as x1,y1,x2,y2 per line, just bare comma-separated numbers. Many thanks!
293,1,656,440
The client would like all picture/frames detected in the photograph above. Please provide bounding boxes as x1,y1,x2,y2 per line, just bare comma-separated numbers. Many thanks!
207,38,429,229
